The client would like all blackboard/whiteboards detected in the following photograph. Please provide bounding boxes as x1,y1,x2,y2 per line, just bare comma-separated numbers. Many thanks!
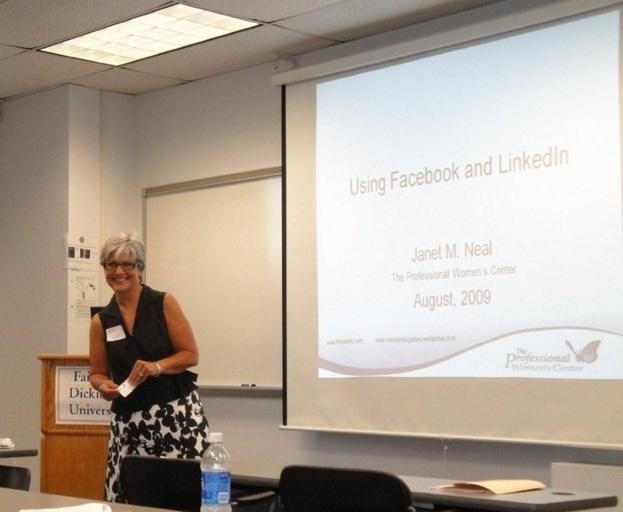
141,165,283,400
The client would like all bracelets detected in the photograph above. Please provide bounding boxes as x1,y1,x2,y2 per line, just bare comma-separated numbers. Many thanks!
155,363,161,378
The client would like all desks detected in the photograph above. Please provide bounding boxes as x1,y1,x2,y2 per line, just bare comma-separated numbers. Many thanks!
0,442,623,512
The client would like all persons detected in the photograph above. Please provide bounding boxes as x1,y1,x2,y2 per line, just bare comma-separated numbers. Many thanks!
89,234,211,505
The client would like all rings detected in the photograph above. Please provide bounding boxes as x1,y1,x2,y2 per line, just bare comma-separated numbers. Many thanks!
102,392,104,395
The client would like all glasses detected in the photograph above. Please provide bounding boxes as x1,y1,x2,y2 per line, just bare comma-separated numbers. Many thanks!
103,260,135,270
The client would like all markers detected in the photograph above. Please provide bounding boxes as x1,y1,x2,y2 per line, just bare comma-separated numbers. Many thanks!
240,384,255,388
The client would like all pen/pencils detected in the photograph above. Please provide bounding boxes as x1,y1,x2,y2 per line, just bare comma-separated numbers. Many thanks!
552,493,575,495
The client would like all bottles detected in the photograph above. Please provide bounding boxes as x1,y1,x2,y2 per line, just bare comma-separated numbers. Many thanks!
199,431,233,512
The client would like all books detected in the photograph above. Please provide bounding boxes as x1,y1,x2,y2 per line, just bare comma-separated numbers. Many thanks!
0,437,14,451
432,478,546,495
